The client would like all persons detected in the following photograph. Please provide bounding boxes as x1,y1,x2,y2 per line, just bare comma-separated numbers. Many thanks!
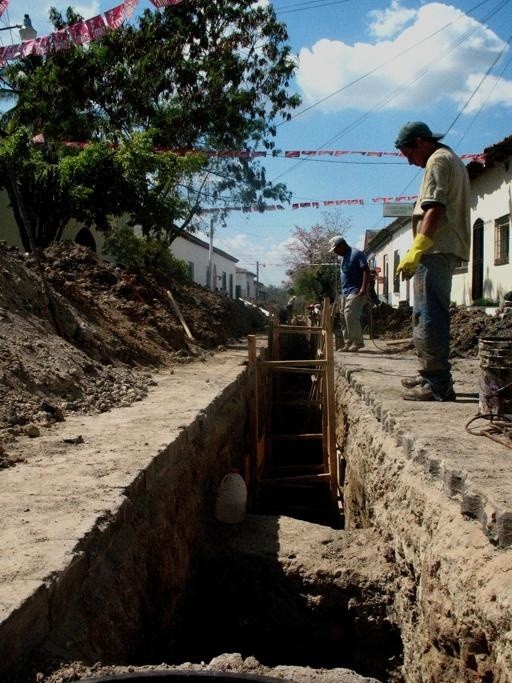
393,121,471,401
328,235,371,353
279,295,322,326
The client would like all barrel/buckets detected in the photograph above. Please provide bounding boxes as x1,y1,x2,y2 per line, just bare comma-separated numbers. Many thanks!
477,336,511,418
214,468,248,524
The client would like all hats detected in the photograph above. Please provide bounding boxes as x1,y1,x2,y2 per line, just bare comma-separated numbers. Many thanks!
328,236,343,252
395,122,444,148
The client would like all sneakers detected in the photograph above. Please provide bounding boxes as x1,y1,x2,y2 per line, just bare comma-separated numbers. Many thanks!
338,340,364,351
402,376,456,401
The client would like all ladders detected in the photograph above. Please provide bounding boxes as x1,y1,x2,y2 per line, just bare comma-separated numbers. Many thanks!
247,297,340,511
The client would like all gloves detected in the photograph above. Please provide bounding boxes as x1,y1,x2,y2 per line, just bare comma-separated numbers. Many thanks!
394,233,433,281
